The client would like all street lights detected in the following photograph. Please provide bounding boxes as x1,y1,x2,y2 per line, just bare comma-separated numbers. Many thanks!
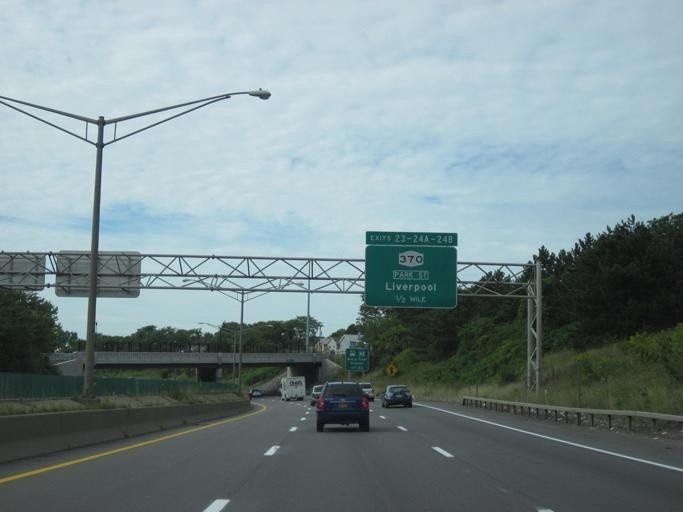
1,87,273,399
180,276,304,397
281,275,344,351
198,320,274,384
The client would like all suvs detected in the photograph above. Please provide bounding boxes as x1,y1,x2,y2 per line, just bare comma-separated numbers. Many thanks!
314,381,369,431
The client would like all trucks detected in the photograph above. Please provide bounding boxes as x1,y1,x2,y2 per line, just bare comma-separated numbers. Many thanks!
279,376,305,401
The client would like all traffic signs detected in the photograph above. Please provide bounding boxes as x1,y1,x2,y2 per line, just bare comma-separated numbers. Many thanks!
345,348,369,371
366,231,456,247
364,246,456,307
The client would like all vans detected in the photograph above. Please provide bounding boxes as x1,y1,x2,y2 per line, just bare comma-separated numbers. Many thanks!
358,382,372,401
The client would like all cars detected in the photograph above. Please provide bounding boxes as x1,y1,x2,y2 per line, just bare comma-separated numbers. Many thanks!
249,388,261,396
311,385,323,403
381,384,412,408
